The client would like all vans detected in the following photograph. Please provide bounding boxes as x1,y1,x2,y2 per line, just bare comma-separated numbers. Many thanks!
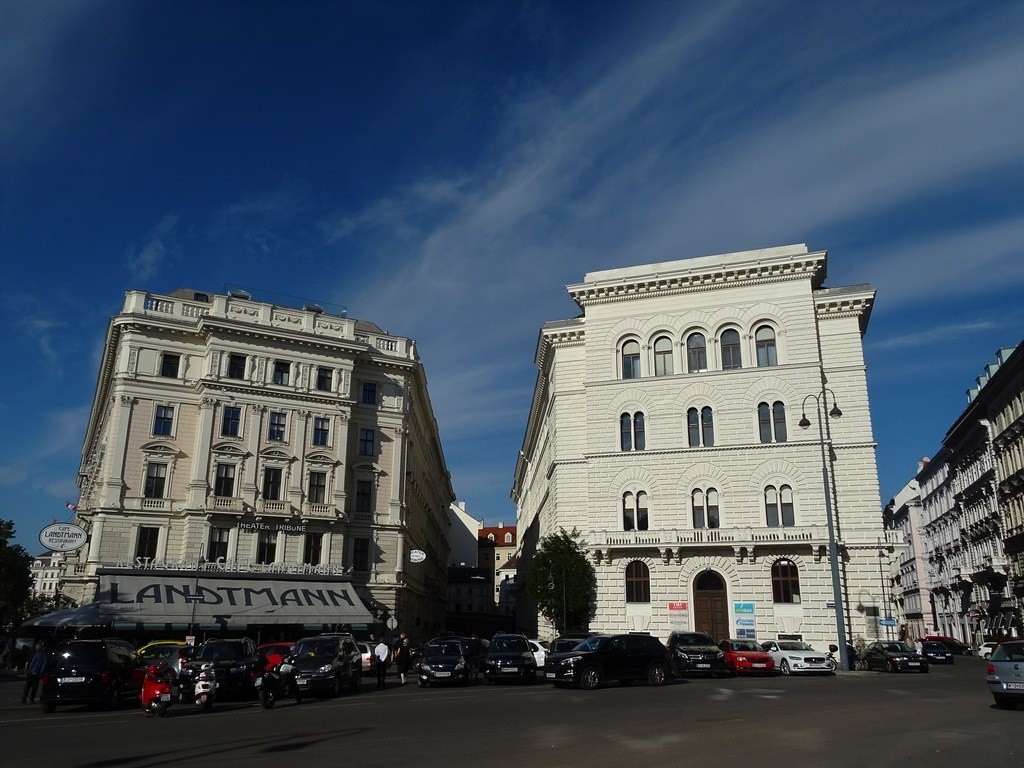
926,636,973,656
288,634,363,698
43,638,149,711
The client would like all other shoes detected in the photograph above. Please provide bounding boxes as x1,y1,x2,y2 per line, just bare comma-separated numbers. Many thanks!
23,702,26,704
401,684,405,686
30,701,34,703
405,682,407,685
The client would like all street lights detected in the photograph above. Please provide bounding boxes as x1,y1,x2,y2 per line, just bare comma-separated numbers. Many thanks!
185,542,207,638
798,388,852,670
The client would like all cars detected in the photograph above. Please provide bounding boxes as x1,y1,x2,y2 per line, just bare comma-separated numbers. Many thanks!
721,640,775,676
924,642,954,664
979,642,997,660
358,642,379,676
526,639,550,667
131,641,187,663
418,639,471,687
862,641,929,673
762,641,833,675
987,643,1024,706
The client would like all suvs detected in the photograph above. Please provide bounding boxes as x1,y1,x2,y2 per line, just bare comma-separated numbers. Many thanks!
540,636,585,650
408,634,481,671
669,630,727,678
241,642,294,701
485,631,537,685
544,634,675,688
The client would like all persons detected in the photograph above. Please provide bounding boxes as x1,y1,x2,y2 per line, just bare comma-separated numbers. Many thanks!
393,634,411,652
374,638,389,687
22,647,47,704
397,638,411,686
914,637,923,655
905,634,915,650
467,633,481,680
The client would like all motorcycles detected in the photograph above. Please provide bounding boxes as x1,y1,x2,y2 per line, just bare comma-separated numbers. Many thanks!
254,653,303,708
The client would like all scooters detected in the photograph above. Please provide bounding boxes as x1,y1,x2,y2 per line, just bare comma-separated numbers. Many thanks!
138,661,173,717
797,639,838,671
846,640,863,671
195,662,219,709
178,657,196,703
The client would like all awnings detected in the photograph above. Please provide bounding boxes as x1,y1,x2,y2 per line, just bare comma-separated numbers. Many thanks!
20,568,375,626
